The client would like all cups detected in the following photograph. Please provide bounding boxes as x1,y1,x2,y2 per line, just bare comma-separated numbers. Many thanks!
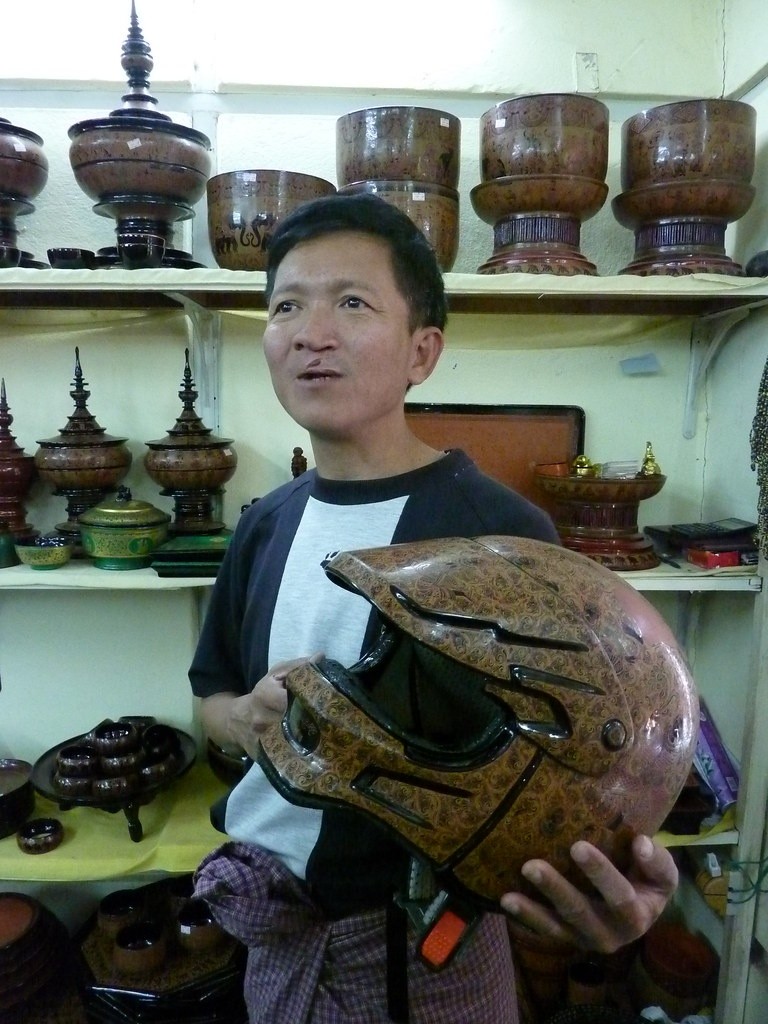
566,958,611,1005
49,714,183,798
16,817,65,854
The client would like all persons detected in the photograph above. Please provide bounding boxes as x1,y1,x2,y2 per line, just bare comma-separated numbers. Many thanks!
187,192,678,1024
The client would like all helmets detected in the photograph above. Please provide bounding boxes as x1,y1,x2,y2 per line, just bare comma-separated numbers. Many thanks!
256,532,699,912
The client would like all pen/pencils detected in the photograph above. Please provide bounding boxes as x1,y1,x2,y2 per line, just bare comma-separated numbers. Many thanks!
658,557,682,569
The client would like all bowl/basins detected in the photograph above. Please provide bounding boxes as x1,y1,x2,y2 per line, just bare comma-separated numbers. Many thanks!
14,544,76,570
619,98,758,193
96,889,150,944
337,179,460,272
478,92,610,185
205,169,337,271
110,922,164,979
334,105,463,191
168,880,224,957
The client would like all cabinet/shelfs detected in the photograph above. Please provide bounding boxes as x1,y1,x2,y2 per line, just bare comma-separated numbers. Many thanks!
0,267,768,1024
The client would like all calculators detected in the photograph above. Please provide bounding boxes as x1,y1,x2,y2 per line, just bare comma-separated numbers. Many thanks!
642,517,759,542
